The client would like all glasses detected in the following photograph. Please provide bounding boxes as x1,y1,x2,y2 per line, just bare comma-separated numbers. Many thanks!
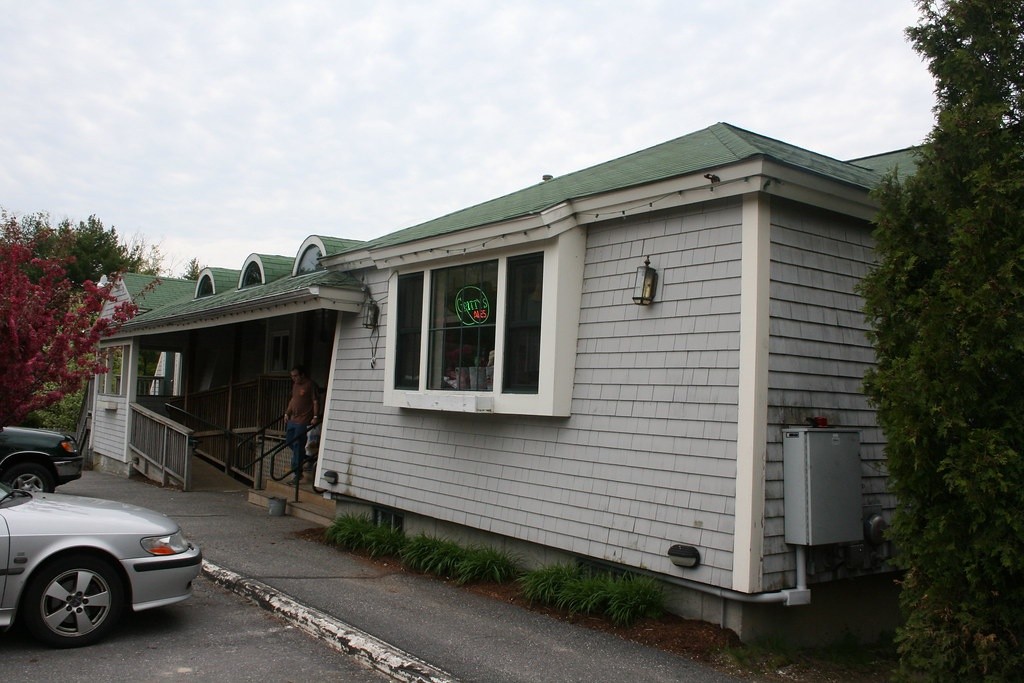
290,374,299,378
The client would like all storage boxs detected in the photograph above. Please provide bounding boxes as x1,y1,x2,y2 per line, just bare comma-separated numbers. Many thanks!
780,426,865,548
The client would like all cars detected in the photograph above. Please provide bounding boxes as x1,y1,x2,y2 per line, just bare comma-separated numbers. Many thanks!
0,424,84,494
0,483,203,648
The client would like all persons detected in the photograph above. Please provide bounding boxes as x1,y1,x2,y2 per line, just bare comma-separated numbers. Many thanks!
283,364,318,484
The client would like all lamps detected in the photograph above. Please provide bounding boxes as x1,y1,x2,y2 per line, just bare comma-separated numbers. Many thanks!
362,298,380,329
631,254,658,306
324,470,338,484
667,544,701,568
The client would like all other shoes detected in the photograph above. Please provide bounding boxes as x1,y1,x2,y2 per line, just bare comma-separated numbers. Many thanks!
303,456,318,470
287,474,304,485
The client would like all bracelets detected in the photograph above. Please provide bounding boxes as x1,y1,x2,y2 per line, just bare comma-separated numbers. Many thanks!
313,416,318,419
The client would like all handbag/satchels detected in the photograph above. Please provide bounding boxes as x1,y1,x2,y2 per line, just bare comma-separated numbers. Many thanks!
304,424,322,455
